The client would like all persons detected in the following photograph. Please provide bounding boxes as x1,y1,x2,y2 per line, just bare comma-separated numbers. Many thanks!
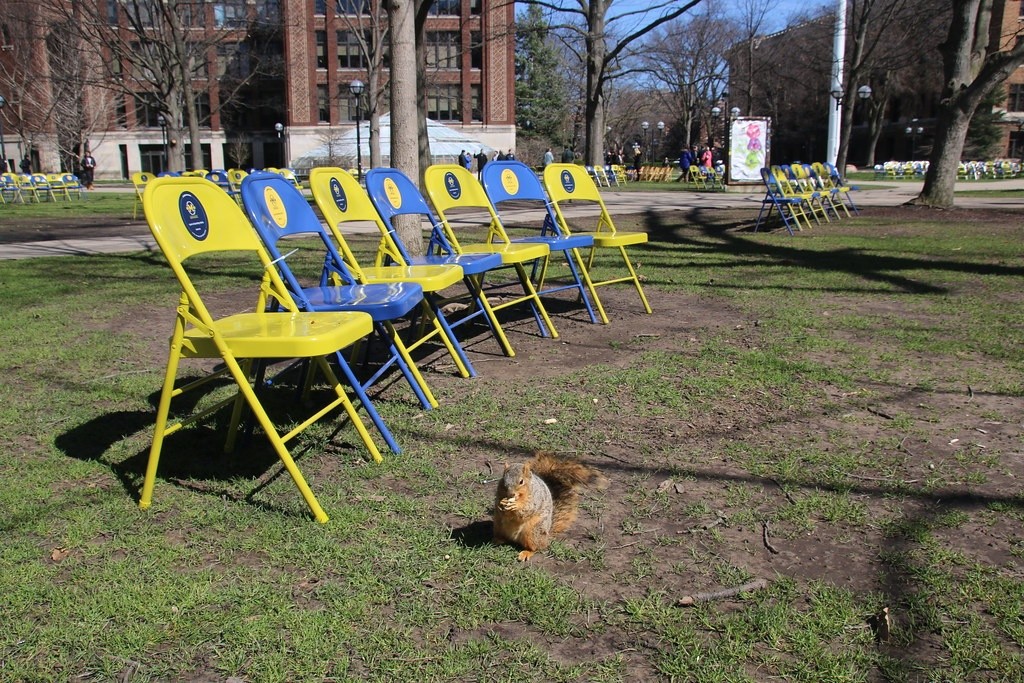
543,147,554,167
605,148,625,165
492,149,515,161
633,147,642,181
662,157,670,167
459,149,469,168
19,155,32,175
562,144,574,163
474,149,487,180
674,143,721,182
466,153,472,171
81,151,96,190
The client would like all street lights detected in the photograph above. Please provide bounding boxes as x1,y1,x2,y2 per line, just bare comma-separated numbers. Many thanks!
711,106,721,147
274,122,284,168
904,117,924,161
349,79,365,182
157,115,167,173
657,121,666,164
641,121,650,163
830,83,872,164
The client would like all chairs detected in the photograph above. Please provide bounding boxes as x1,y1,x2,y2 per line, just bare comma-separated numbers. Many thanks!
755,162,860,234
873,158,1022,182
0,173,87,204
131,163,725,524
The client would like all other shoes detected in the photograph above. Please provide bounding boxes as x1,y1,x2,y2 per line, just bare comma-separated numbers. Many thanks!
87,184,93,190
